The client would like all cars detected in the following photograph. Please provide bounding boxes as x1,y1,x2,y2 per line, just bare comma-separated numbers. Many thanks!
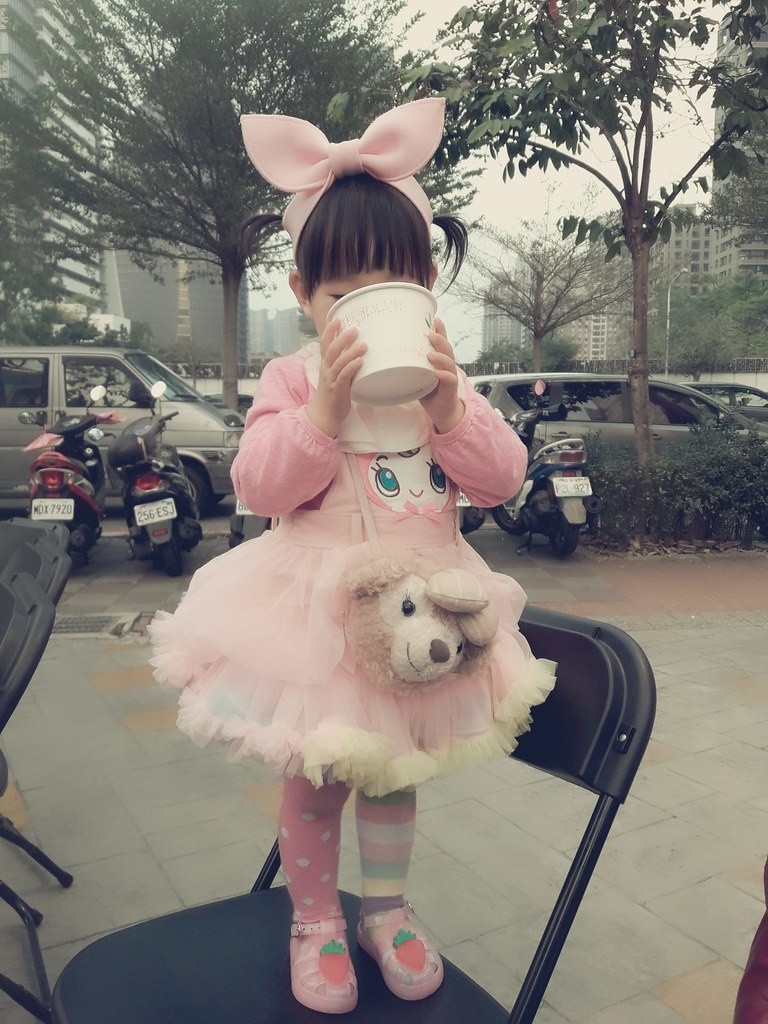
673,379,768,425
465,372,768,469
204,393,256,414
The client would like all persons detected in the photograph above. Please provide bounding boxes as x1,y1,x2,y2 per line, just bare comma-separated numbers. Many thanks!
146,163,560,1014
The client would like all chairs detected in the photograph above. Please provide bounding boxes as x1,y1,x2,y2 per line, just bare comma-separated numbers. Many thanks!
0,519,72,1024
49,603,656,1024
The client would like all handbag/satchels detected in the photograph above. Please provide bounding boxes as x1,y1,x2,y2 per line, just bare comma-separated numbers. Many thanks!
342,543,500,695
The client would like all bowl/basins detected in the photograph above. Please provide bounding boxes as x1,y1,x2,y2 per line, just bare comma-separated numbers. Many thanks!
325,282,438,408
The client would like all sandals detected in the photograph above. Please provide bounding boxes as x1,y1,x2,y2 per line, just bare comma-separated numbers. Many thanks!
289,918,359,1015
358,902,444,1000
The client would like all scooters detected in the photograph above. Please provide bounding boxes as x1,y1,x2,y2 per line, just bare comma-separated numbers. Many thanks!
457,489,487,533
224,413,281,550
16,384,127,568
491,379,605,555
86,381,205,576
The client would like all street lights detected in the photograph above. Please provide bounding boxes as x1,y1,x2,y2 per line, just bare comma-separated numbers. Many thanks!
665,267,689,378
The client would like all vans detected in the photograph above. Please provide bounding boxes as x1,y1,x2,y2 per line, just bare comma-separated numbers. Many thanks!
0,345,245,515
